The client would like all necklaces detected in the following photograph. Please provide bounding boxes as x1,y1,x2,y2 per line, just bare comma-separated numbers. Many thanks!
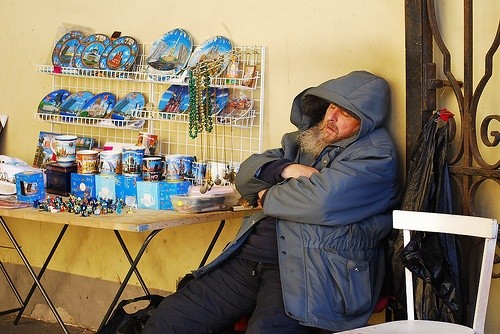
189,71,198,139
196,67,212,133
197,70,237,194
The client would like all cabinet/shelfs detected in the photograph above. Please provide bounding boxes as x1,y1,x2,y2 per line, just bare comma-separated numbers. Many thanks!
33,26,265,187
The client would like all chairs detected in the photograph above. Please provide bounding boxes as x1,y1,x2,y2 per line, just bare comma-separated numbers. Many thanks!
333,210,499,334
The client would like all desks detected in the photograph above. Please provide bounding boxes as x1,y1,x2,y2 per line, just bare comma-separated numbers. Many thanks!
0,188,264,334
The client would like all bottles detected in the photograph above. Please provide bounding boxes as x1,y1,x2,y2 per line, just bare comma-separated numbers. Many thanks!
110,92,147,128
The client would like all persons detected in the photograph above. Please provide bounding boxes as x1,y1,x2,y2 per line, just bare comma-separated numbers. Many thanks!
142,71,405,334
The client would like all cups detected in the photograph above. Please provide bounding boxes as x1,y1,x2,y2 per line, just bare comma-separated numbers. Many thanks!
164,155,184,182
136,132,159,158
181,154,197,180
191,162,206,186
98,150,122,176
122,148,145,177
50,135,77,163
142,155,167,181
76,150,99,175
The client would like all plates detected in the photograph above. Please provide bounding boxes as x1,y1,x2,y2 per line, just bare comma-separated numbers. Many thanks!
98,36,139,77
37,89,70,120
52,31,87,74
73,34,113,76
0,181,17,195
157,84,255,124
144,28,233,83
58,91,94,122
76,92,116,124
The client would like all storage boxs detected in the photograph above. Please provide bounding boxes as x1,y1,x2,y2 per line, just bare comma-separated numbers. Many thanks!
95,173,121,204
15,170,46,204
71,173,101,200
46,161,78,197
159,179,191,210
137,180,162,210
